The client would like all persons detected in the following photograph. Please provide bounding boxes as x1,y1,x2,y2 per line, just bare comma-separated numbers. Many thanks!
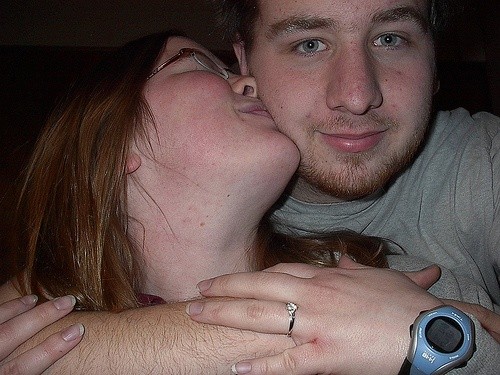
0,0,500,375
0,28,500,375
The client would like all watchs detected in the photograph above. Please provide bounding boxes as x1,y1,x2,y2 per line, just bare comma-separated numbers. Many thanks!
397,305,477,375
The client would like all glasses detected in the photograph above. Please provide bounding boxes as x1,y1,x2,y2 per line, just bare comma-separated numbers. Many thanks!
139,48,231,84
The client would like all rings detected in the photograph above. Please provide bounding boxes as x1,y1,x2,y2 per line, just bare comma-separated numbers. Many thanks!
286,301,298,335
